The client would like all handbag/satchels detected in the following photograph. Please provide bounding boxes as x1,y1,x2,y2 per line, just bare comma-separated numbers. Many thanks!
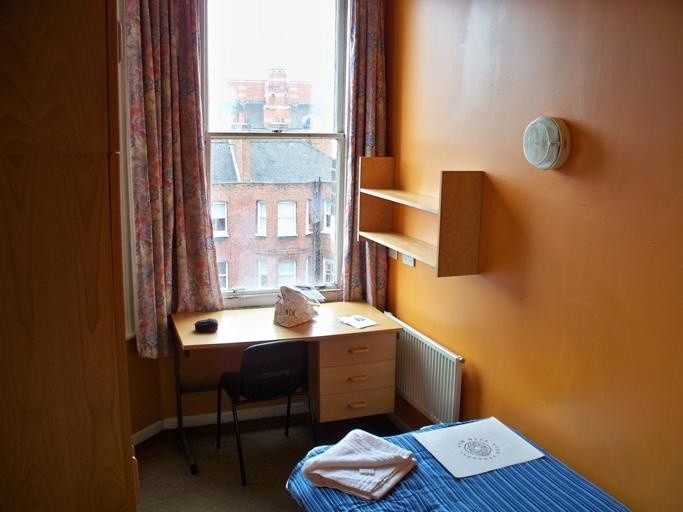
274,286,320,327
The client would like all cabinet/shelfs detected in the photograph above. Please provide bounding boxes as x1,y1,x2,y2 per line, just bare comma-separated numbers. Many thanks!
355,155,485,277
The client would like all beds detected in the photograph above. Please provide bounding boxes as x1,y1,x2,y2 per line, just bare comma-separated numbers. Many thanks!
284,415,634,512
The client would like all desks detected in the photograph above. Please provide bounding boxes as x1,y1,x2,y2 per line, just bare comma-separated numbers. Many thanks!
170,300,404,476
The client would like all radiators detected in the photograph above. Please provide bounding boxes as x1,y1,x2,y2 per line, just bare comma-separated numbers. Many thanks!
383,311,464,428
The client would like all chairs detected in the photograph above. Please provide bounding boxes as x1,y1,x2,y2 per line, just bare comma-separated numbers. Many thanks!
214,339,317,488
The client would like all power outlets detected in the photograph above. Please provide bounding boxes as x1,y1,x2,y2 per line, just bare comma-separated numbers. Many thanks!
402,254,414,267
388,248,397,259
